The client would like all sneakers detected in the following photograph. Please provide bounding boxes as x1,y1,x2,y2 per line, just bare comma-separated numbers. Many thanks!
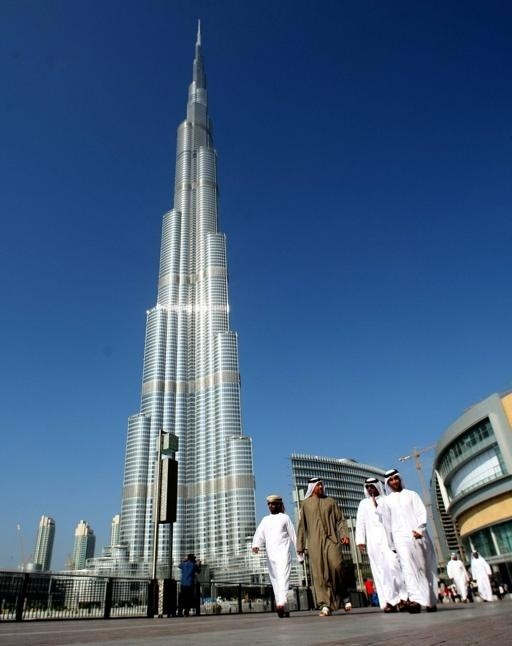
275,601,438,619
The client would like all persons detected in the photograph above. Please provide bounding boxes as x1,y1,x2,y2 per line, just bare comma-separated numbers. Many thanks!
446,552,474,603
251,495,305,618
362,578,374,607
471,550,494,602
296,477,352,616
382,469,439,614
178,554,201,617
355,477,406,613
